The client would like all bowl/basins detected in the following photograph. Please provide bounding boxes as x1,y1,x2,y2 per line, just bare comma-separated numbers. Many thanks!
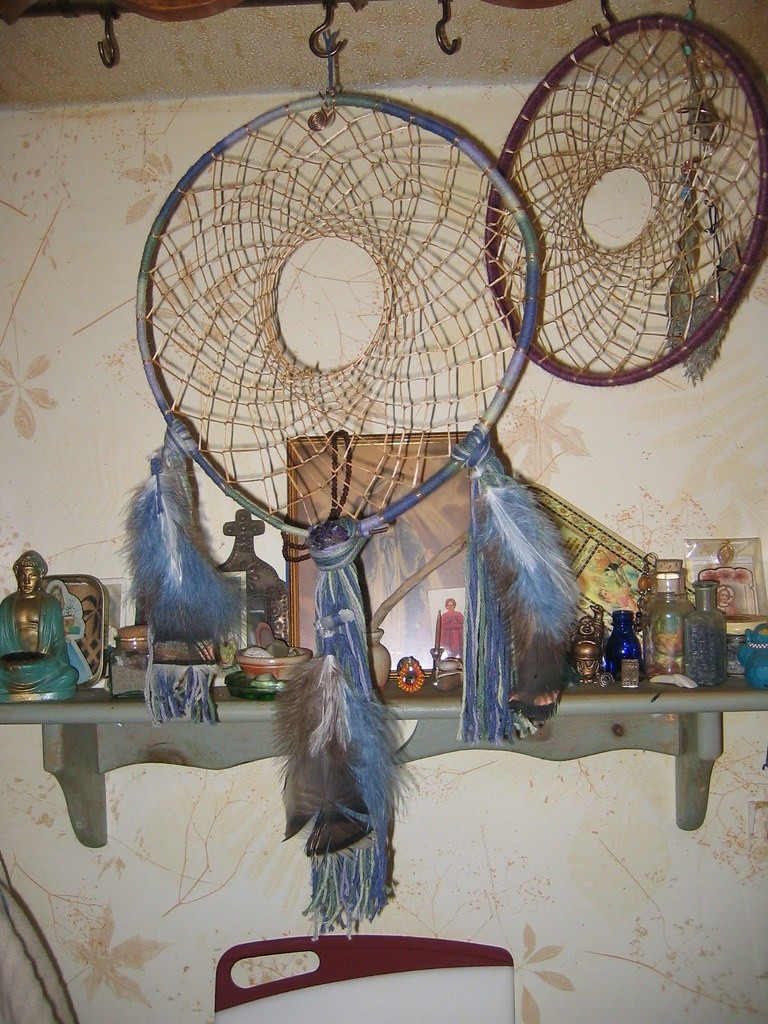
236,647,313,680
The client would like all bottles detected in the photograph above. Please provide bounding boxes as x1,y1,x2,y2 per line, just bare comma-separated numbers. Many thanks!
642,573,695,678
366,629,391,691
685,580,727,687
606,610,642,682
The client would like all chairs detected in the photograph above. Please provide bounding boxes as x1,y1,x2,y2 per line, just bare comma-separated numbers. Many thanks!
213,935,515,1024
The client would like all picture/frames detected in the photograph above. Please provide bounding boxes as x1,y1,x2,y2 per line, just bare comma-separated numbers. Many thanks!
286,431,470,681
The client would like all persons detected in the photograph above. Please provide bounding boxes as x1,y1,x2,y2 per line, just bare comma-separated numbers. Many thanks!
0,550,67,713
